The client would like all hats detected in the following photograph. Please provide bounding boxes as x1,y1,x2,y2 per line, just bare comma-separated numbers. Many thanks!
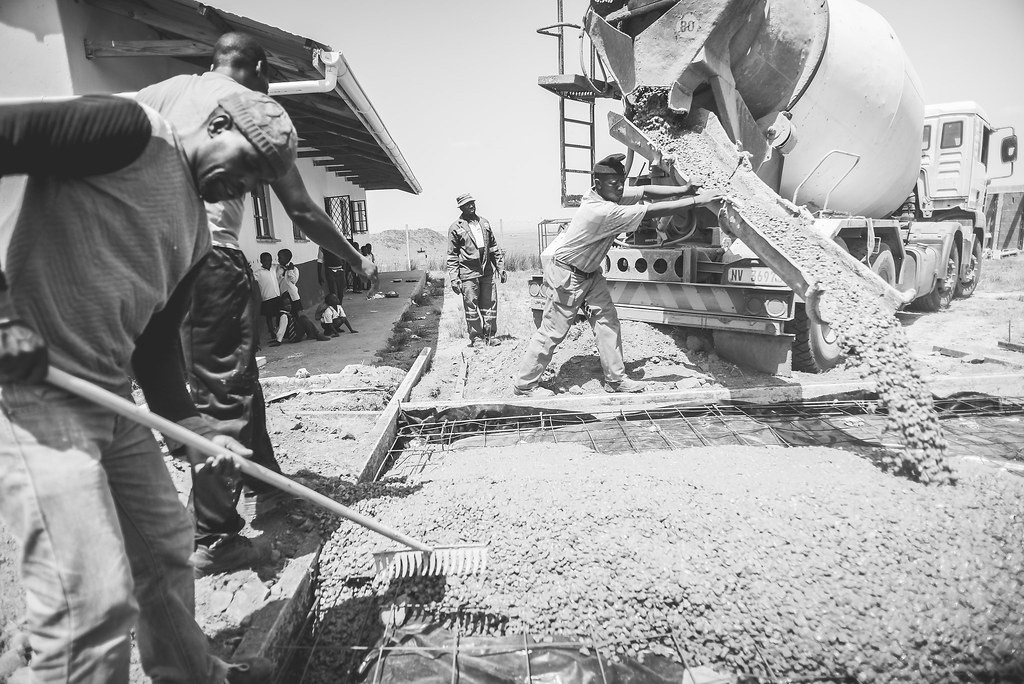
593,153,627,175
218,90,299,177
456,193,475,208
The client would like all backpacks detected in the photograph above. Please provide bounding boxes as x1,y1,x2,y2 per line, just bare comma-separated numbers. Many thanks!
314,303,341,320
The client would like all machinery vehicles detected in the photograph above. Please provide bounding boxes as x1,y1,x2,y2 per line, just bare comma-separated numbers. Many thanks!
528,0,1018,381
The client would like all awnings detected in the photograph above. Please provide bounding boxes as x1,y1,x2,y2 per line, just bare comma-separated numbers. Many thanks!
80,0,423,195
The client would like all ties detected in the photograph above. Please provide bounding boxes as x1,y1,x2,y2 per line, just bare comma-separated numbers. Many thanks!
281,266,288,276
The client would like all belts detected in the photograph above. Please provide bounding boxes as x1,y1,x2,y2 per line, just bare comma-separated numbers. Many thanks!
551,256,594,279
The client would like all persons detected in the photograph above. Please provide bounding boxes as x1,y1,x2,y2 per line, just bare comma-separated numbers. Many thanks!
351,242,362,293
315,246,345,305
275,249,301,321
313,294,359,338
514,154,727,397
251,252,331,347
133,28,376,572
0,90,298,684
447,193,507,348
361,243,375,290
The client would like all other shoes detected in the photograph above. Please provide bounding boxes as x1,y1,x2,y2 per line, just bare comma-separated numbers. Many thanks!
604,377,648,393
472,336,484,352
191,534,272,578
486,335,501,346
222,653,274,684
245,477,317,514
514,384,555,397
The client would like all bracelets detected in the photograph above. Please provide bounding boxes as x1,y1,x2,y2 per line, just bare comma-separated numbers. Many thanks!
691,197,697,206
0,315,34,332
